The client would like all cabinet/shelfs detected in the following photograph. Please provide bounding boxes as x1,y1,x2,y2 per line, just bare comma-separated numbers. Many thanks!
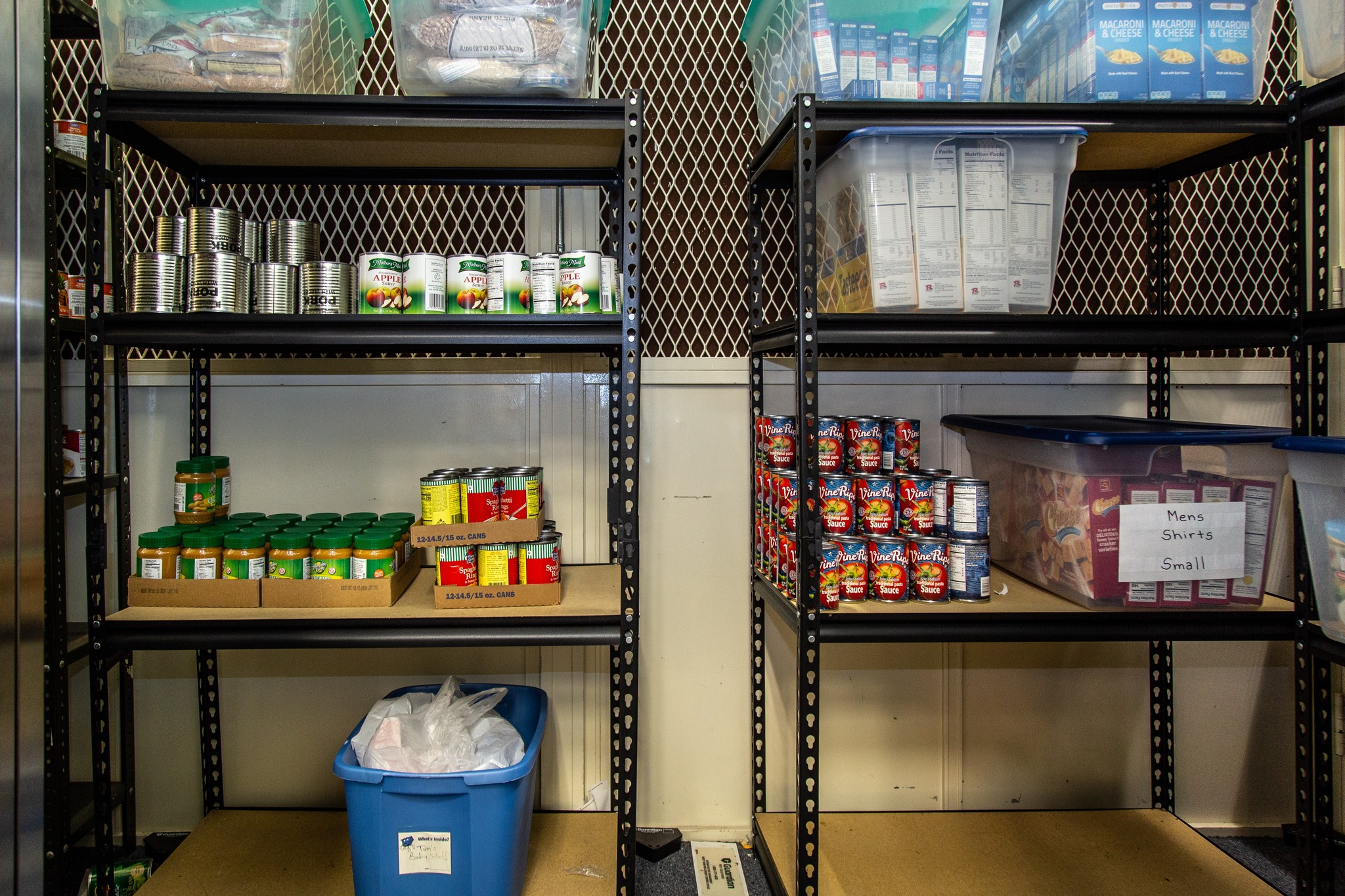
83,89,642,896
1297,73,1345,896
750,91,1317,896
42,0,134,896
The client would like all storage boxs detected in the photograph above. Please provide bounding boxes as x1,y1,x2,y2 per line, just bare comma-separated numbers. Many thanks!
737,0,1345,315
409,509,549,548
433,567,561,609
127,572,270,609
259,548,422,608
334,682,549,896
939,414,1298,610
93,0,610,100
1271,435,1345,643
